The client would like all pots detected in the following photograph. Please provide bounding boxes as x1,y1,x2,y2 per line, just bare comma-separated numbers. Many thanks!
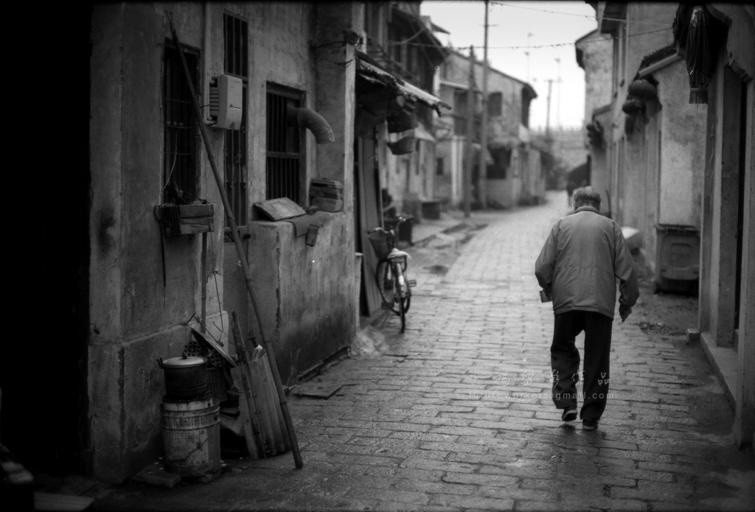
157,352,213,398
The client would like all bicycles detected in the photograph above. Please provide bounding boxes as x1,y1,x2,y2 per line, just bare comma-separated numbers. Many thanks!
366,216,417,333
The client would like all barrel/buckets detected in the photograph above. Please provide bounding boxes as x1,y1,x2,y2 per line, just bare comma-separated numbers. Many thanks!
160,404,223,483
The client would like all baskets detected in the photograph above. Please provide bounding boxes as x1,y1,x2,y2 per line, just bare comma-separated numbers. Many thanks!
370,232,398,257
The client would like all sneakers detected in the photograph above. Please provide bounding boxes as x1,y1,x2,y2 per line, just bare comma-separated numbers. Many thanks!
561,407,598,430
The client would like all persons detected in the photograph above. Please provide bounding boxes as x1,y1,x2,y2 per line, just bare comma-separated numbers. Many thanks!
534,184,641,431
